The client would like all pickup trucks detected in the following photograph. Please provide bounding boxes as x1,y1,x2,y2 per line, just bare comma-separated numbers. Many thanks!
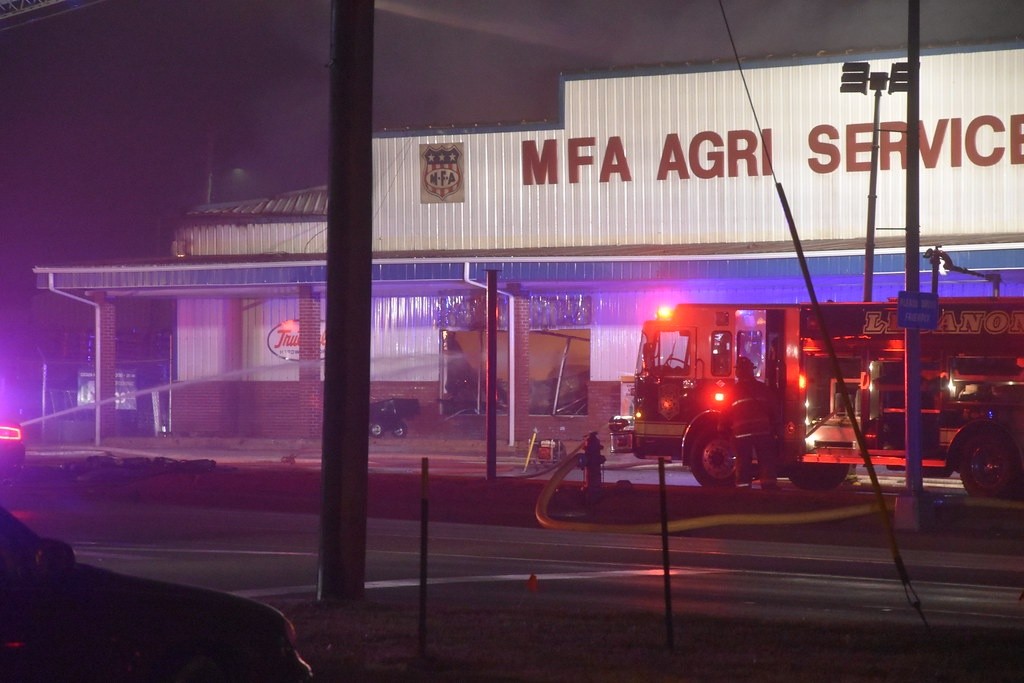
370,397,421,437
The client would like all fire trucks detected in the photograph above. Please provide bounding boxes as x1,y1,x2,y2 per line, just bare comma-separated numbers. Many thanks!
608,293,1024,499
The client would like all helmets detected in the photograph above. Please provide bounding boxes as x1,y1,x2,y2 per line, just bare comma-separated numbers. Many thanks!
733,358,757,369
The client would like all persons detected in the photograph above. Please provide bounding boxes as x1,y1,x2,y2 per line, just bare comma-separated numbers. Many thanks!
718,356,782,504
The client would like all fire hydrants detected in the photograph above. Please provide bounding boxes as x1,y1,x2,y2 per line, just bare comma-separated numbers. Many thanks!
577,432,607,507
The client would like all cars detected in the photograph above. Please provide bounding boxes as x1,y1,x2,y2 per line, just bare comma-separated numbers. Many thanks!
1,505,314,682
